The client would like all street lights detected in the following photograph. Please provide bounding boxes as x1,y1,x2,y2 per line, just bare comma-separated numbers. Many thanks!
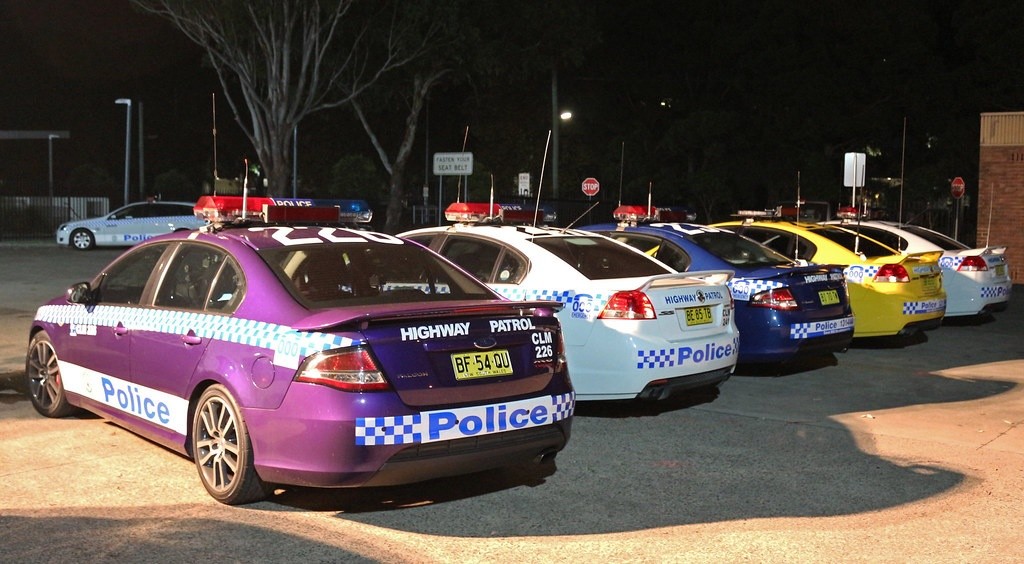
115,96,132,208
48,134,64,234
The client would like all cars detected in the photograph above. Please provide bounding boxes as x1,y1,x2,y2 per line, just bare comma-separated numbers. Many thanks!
709,209,949,343
56,191,208,251
386,199,740,408
811,218,1013,323
576,203,857,376
25,187,576,506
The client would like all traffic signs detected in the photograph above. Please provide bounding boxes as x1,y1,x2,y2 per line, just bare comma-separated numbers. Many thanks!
433,152,472,175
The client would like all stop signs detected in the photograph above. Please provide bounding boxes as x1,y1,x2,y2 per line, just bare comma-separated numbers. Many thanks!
951,177,965,200
581,178,600,197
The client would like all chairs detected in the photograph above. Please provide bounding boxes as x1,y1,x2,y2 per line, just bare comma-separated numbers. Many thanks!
352,272,381,298
155,276,175,306
296,268,337,303
